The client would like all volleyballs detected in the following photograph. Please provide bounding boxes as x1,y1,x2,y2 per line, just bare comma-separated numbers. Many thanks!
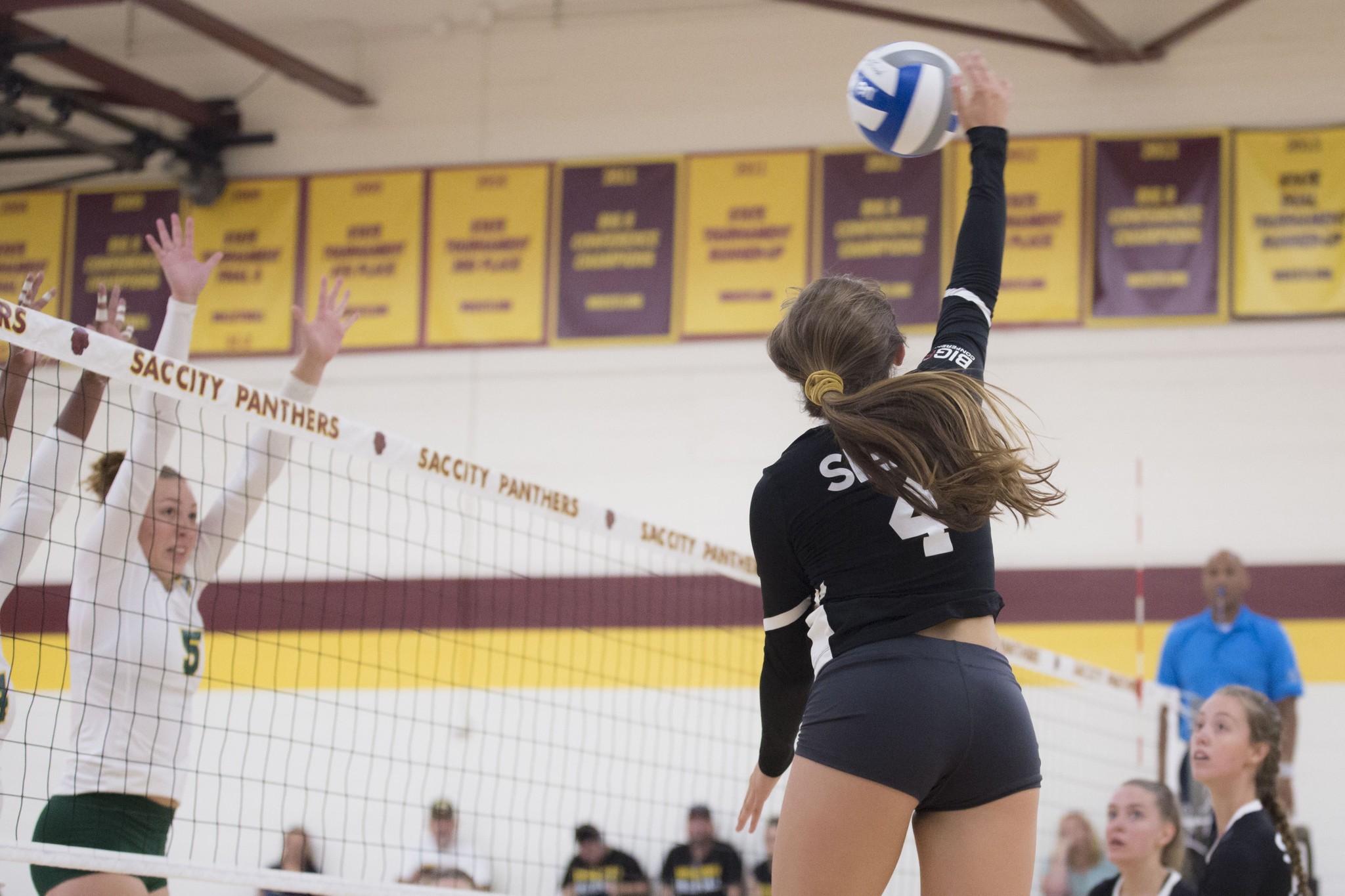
845,40,969,158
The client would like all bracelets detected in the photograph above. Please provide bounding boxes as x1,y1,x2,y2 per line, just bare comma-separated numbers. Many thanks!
1279,762,1294,781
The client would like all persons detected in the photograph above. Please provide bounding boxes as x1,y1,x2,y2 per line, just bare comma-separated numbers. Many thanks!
559,825,652,896
27,211,360,896
733,52,1043,896
1151,552,1306,844
1040,683,1313,896
745,817,781,896
256,828,326,896
393,800,494,891
0,264,137,732
655,806,744,896
